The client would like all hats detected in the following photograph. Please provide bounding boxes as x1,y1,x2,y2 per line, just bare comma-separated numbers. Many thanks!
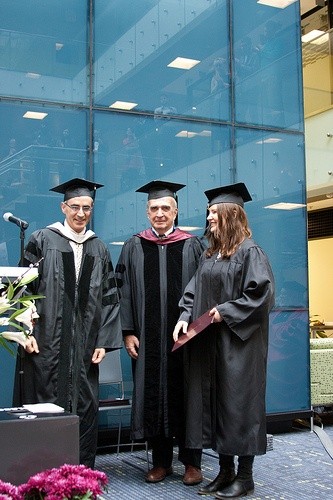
49,178,104,228
200,183,253,240
135,180,187,228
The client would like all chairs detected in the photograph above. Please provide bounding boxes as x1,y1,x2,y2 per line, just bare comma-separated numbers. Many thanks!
97,348,149,471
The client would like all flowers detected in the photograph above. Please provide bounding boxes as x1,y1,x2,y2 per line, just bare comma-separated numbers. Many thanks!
0,255,44,361
0,463,109,500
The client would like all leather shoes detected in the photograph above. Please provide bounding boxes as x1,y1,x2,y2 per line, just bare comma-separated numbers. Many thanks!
183,465,202,484
147,465,172,481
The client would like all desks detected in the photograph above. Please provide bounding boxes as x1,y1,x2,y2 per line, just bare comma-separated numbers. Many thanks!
0,399,80,487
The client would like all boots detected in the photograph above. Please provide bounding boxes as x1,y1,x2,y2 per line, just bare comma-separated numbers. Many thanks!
215,456,255,500
198,454,236,494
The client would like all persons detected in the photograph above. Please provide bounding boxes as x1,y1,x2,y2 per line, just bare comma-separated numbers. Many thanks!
211,36,262,153
82,127,110,200
114,181,209,486
172,182,275,500
12,178,123,469
4,125,79,202
254,21,284,135
118,95,177,189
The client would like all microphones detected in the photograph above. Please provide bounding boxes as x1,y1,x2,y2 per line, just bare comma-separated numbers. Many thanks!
3,212,28,229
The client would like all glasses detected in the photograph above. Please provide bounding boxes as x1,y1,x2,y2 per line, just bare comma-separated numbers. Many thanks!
64,202,93,214
